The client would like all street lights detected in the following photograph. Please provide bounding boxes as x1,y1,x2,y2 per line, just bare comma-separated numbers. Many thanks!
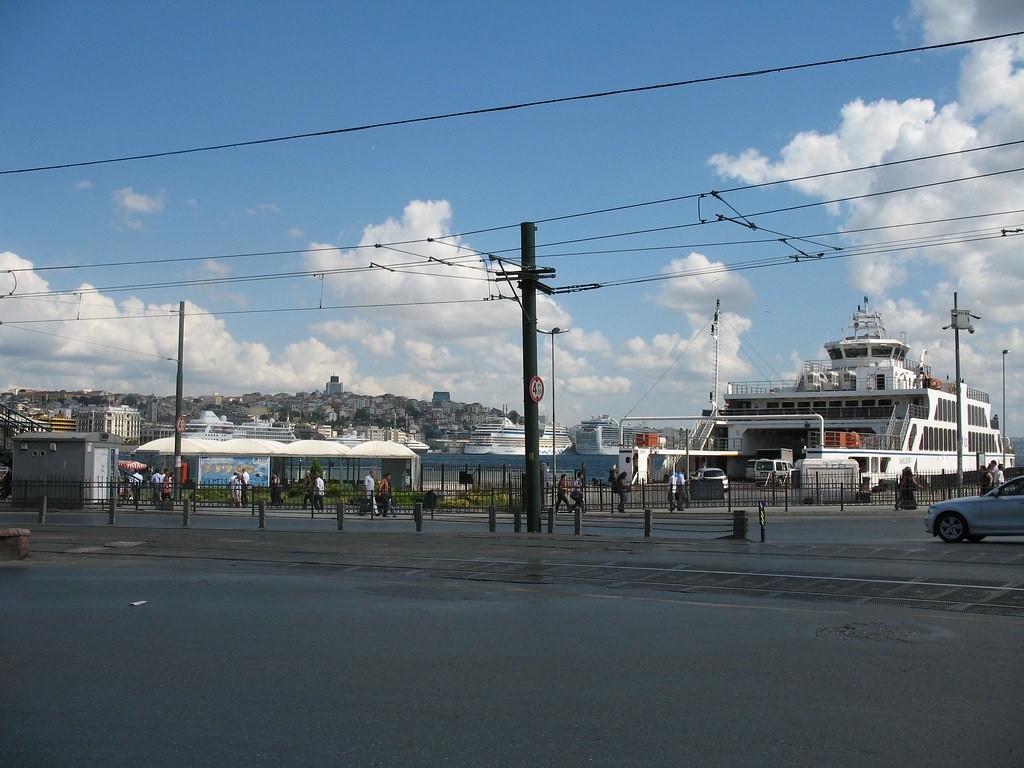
550,327,560,486
1002,349,1008,468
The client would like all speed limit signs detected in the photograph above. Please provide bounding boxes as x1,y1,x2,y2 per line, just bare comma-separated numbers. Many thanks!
176,417,186,433
529,376,544,403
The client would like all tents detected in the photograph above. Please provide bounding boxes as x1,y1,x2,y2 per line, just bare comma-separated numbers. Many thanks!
118,460,146,470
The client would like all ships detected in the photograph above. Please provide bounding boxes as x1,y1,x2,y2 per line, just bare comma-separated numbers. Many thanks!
464,413,661,456
170,414,301,443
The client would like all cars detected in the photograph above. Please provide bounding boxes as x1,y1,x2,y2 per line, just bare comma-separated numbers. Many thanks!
691,467,729,490
924,476,1024,543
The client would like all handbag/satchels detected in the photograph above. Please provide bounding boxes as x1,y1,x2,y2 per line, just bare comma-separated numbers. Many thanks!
570,490,583,503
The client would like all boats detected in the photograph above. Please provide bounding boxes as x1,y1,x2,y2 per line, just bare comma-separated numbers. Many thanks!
664,294,1016,490
324,424,430,455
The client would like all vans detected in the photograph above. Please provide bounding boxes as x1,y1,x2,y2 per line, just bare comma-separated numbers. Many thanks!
746,458,794,487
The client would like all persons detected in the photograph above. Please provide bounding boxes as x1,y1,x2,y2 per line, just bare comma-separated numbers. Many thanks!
994,464,1007,495
360,469,381,516
150,468,175,505
993,414,998,420
608,464,618,481
118,471,134,504
555,474,574,514
912,367,928,388
270,474,285,508
376,473,398,518
229,468,250,507
616,472,629,513
302,473,324,510
989,460,998,476
980,465,993,496
667,467,681,511
570,472,588,514
131,469,143,504
895,467,923,510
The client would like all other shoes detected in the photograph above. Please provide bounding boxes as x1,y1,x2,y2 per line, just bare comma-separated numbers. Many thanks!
358,513,367,516
393,516,398,518
376,514,382,517
669,505,677,510
677,508,685,511
382,515,391,517
555,514,559,515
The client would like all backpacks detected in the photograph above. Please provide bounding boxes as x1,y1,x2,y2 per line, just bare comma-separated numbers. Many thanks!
611,480,624,493
